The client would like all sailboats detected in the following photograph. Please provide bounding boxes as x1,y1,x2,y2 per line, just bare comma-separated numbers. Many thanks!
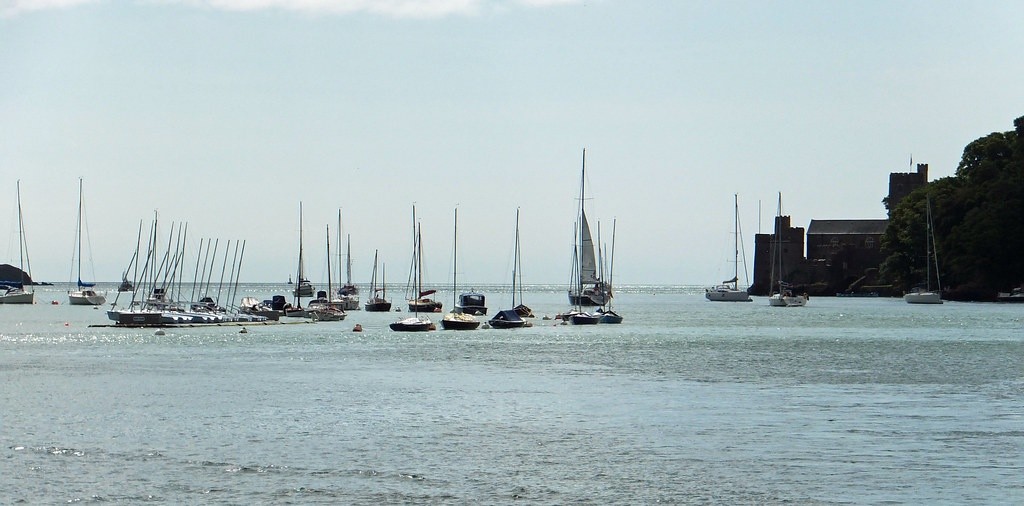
565,146,613,306
703,192,753,302
0,179,35,305
767,192,810,306
67,176,107,306
107,201,625,332
902,193,944,304
